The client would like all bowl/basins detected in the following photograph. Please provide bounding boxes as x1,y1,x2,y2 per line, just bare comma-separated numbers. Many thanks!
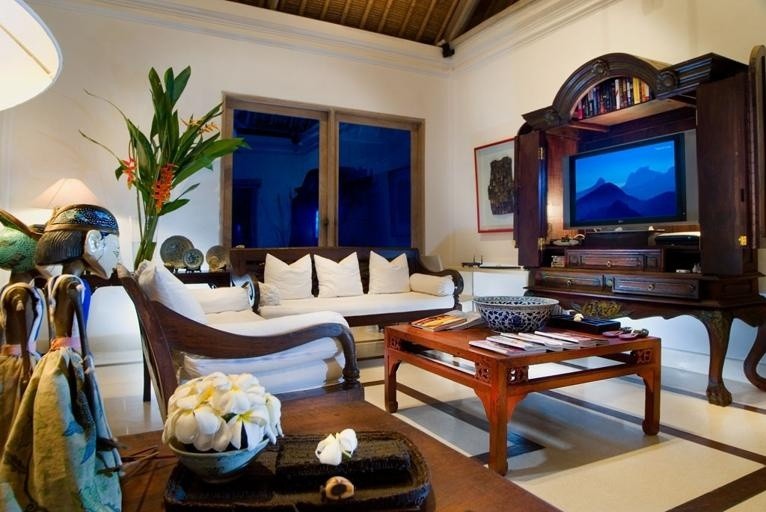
473,295,559,334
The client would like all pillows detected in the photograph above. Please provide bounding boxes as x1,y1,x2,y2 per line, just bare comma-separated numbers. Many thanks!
134,253,208,330
313,249,365,299
366,249,413,297
263,252,314,301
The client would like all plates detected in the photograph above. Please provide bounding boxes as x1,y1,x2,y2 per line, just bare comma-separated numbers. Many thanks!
160,235,194,268
183,247,204,268
205,245,226,269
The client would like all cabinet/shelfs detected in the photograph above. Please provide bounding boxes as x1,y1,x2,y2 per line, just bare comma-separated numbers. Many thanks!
513,45,766,408
454,262,531,312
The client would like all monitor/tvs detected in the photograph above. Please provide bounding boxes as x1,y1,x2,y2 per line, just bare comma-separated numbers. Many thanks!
562,125,700,234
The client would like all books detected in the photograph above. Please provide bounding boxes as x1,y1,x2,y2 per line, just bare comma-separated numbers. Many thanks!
410,313,467,334
576,74,653,120
470,328,613,357
436,309,485,331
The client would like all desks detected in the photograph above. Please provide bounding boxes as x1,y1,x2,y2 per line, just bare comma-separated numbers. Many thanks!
80,264,232,403
109,389,565,511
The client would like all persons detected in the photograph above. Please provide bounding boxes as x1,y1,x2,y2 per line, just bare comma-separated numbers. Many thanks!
0,204,123,511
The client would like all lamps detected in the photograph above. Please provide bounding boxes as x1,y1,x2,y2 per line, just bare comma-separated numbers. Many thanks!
436,39,459,62
0,1,65,112
27,174,106,218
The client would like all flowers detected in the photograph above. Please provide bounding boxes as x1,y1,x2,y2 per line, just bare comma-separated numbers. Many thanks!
79,65,246,271
159,371,286,455
314,425,359,468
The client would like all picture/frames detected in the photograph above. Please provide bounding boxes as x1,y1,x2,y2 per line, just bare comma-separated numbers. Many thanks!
472,136,517,234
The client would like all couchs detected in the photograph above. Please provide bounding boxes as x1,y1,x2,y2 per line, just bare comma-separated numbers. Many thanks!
223,243,466,362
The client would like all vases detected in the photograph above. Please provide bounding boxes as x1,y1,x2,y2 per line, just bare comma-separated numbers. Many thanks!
122,213,161,272
166,435,271,486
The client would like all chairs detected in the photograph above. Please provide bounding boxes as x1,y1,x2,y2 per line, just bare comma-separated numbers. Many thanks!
113,259,365,428
